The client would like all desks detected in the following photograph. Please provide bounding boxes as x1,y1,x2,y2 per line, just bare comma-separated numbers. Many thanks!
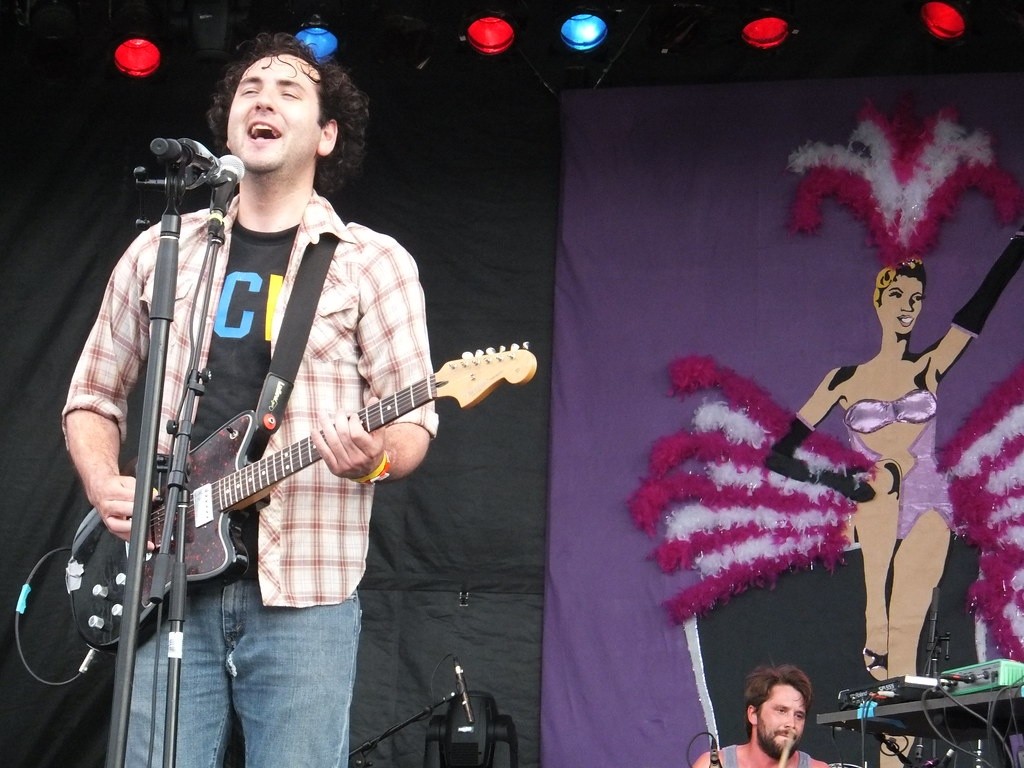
817,687,1024,768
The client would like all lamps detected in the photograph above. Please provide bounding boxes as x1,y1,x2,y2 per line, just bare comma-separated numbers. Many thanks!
452,0,515,53
107,2,164,75
549,1,610,51
291,0,340,60
917,0,971,40
731,0,790,48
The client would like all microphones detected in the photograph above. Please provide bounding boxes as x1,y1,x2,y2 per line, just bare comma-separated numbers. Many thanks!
208,154,248,217
454,663,475,723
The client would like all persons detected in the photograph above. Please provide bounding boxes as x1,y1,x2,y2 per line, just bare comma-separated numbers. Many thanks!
687,662,831,768
59,30,439,768
762,227,1024,767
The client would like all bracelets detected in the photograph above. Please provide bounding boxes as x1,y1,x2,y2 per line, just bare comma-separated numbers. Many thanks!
348,451,391,486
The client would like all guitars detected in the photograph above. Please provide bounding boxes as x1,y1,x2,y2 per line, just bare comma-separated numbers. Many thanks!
64,339,540,650
